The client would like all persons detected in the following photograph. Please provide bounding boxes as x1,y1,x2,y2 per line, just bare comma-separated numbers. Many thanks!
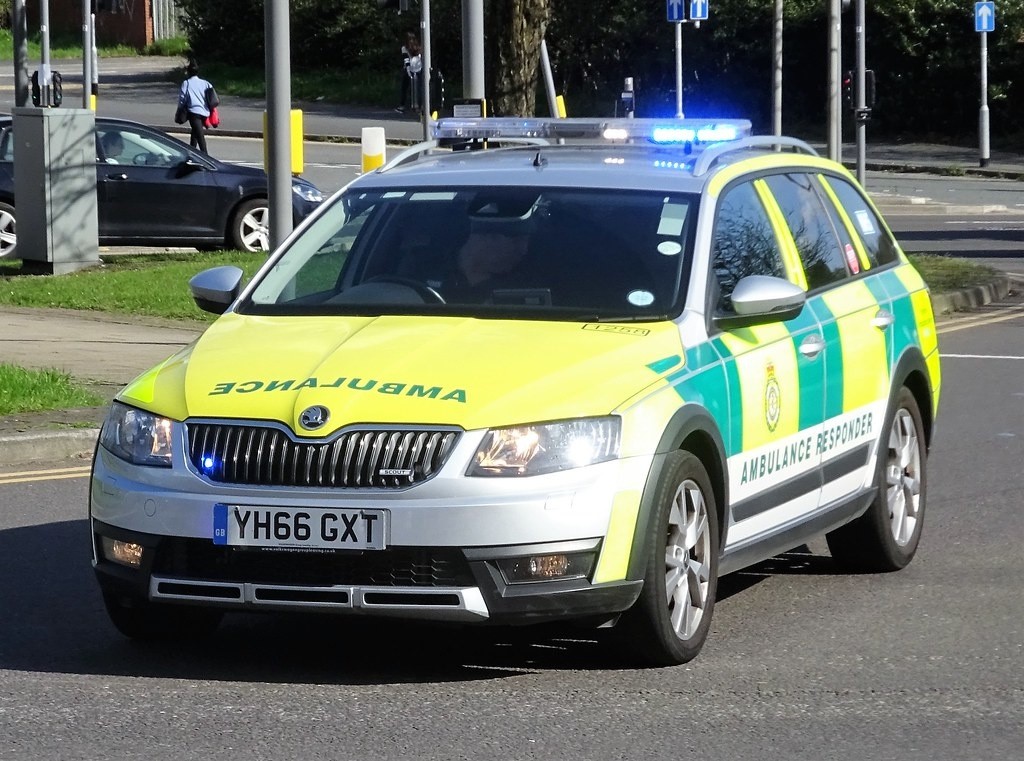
178,63,213,155
101,132,124,157
404,199,530,303
394,32,422,113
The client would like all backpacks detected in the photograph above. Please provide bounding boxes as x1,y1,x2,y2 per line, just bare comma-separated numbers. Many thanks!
409,54,423,74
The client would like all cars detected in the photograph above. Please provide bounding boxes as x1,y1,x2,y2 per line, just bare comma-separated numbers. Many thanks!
1,111,327,262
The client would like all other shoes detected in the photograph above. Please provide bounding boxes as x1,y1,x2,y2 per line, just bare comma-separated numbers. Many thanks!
393,107,403,112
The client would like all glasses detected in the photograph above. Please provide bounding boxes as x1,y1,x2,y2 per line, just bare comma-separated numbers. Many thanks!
113,141,124,150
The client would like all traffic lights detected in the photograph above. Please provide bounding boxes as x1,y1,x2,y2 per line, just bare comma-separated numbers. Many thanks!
842,71,855,112
30,70,40,107
52,72,63,106
865,69,877,108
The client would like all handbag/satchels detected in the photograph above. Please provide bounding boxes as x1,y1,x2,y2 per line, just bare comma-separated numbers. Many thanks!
205,87,219,111
175,94,192,125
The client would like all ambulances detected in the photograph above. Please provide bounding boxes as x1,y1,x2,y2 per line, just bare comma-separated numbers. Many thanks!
86,114,946,665
